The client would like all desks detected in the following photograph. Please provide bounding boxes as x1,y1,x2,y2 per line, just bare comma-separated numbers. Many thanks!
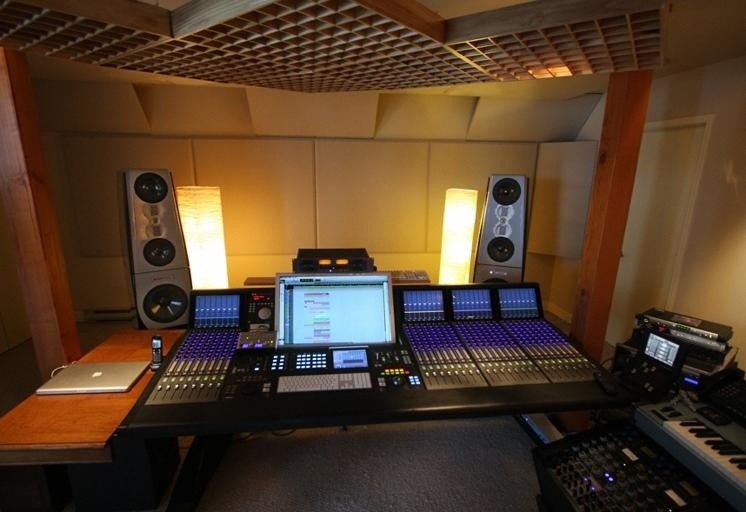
0,329,186,512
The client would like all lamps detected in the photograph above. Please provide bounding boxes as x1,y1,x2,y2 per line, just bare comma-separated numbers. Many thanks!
439,188,479,284
175,184,229,290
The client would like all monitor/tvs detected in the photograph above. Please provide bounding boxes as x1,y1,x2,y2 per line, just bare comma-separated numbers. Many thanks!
275,273,396,345
643,332,680,366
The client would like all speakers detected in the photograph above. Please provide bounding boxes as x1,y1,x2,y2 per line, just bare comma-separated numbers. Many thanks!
122,167,193,329
472,173,530,282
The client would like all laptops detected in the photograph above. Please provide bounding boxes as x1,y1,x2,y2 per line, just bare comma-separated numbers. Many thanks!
35,360,151,395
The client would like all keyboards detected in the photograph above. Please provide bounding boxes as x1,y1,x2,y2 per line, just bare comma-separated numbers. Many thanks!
276,372,372,393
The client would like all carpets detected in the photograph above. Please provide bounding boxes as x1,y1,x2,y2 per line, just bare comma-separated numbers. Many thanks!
194,415,542,512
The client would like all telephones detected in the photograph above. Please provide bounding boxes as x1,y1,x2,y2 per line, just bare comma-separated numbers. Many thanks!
149,335,166,371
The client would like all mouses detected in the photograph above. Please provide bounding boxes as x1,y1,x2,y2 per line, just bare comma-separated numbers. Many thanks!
392,376,404,386
